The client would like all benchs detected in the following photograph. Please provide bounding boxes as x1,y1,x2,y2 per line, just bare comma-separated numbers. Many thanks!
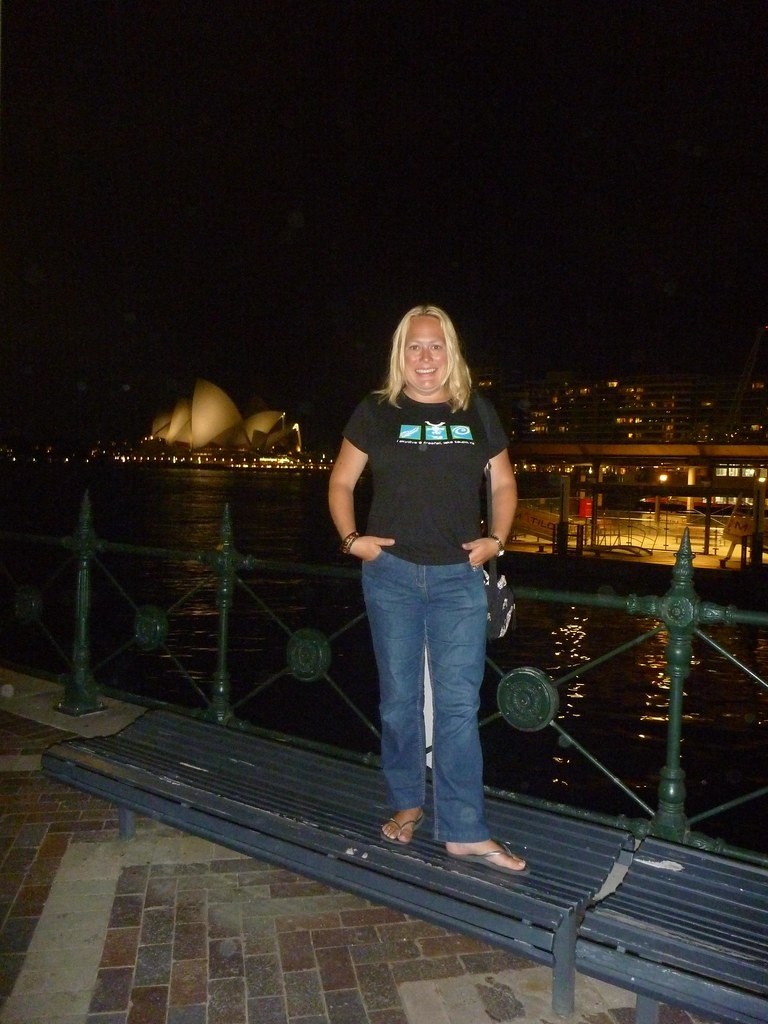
39,708,635,1014
574,836,768,1024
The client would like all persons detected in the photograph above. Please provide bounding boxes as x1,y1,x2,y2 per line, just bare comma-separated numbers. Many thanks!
328,306,528,874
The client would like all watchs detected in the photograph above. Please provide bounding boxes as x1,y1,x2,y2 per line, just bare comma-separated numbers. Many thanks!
489,534,505,558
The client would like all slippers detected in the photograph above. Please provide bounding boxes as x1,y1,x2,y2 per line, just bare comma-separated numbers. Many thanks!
442,837,530,878
380,811,426,846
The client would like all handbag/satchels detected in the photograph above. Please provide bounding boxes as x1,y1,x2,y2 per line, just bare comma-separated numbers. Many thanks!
484,557,517,643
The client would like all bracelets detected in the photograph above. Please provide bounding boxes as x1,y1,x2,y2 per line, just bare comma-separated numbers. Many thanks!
340,532,361,555
